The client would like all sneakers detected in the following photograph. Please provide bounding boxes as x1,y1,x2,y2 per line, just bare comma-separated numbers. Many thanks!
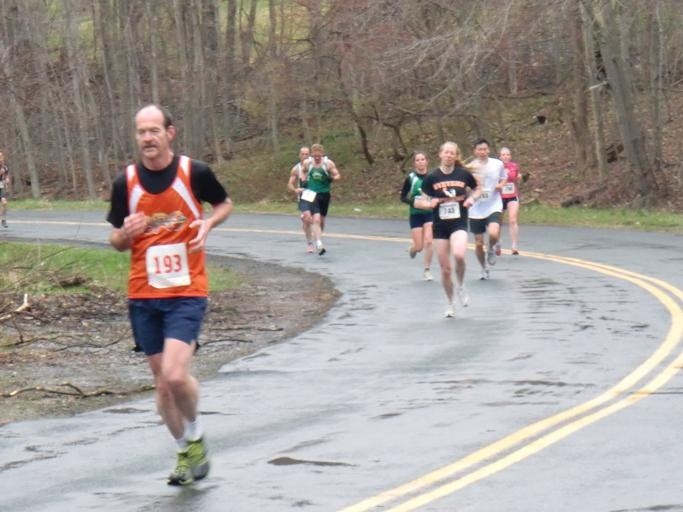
422,267,433,281
306,241,326,255
185,435,210,481
165,450,193,485
481,270,489,280
487,244,519,265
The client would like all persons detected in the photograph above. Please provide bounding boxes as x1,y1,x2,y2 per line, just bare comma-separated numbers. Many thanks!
302,144,340,254
0,151,10,226
400,152,433,281
288,146,316,252
421,140,481,318
465,138,507,281
106,105,232,485
495,146,520,257
